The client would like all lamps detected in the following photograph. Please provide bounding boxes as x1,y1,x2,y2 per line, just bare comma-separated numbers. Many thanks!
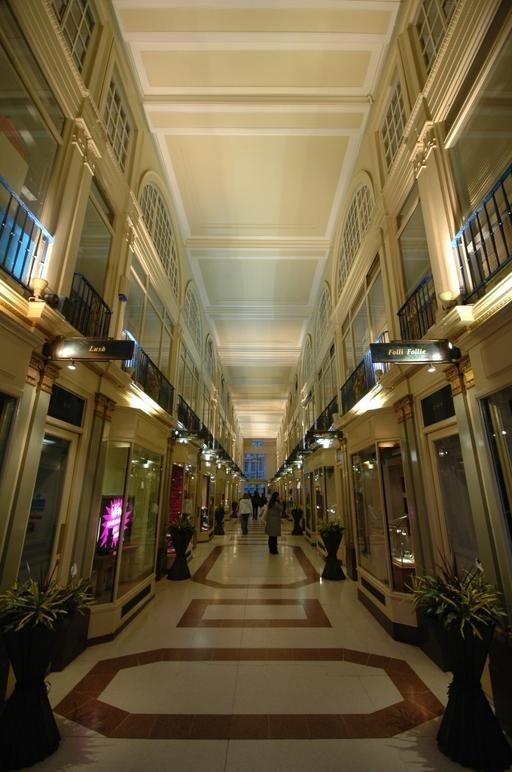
65,360,78,372
423,361,441,377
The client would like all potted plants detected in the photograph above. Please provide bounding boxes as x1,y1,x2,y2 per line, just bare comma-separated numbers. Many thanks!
1,561,104,771
318,518,349,582
405,538,512,771
163,510,198,580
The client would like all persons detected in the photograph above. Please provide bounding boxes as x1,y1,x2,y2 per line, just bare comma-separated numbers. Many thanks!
260,492,267,517
238,492,252,534
252,490,259,521
265,492,286,554
